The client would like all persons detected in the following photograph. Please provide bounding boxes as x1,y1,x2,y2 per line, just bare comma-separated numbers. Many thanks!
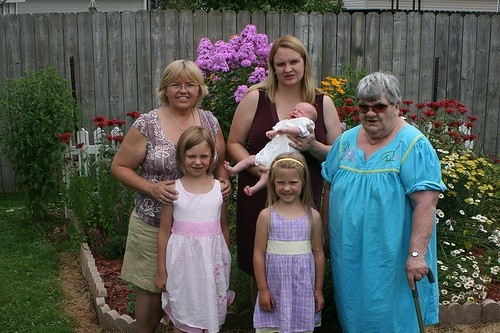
226,37,343,277
110,61,232,331
225,103,318,197
252,153,325,333
323,72,447,333
155,126,231,333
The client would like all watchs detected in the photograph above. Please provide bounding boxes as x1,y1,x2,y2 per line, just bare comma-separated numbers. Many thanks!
408,251,426,259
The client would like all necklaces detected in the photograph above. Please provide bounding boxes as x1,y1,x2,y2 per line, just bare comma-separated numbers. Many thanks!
362,135,386,146
165,110,199,132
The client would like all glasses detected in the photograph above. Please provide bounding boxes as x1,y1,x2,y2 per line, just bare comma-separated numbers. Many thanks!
167,81,199,89
358,103,392,113
